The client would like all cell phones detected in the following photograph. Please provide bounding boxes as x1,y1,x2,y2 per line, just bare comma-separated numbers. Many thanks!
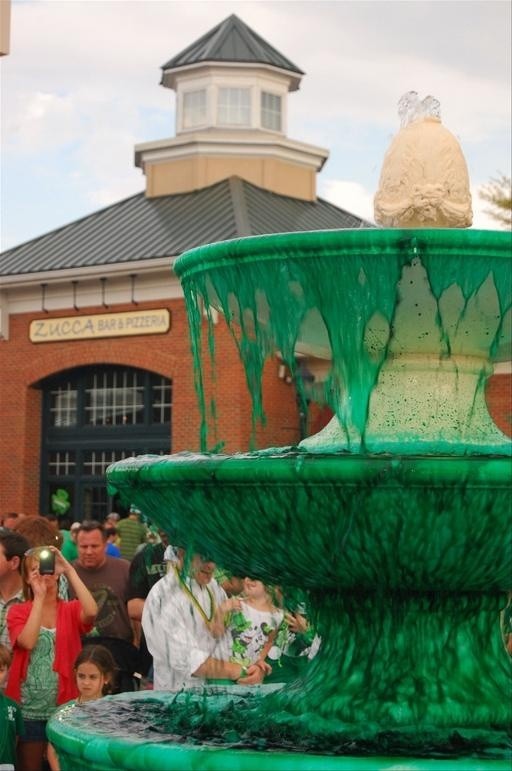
39,551,55,575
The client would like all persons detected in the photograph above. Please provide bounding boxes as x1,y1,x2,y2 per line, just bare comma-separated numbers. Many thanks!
1,512,322,771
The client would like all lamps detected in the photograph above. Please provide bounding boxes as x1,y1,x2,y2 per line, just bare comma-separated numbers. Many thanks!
72,281,79,312
41,284,49,313
129,273,139,306
100,277,109,308
